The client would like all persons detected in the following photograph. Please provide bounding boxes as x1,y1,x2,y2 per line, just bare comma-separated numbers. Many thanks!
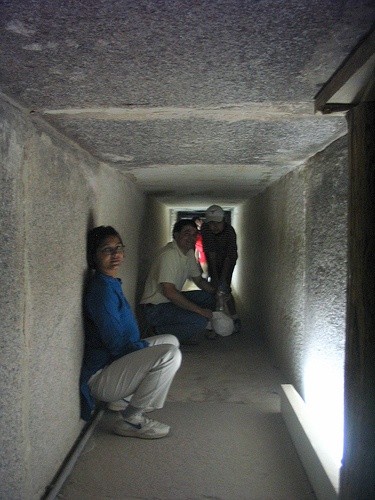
138,219,217,348
202,204,242,340
192,216,209,282
80,226,182,439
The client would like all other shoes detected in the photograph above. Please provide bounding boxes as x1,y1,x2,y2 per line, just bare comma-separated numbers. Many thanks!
233,318,241,332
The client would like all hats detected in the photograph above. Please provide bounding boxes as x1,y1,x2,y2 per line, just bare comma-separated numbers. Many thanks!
210,312,233,336
205,204,225,223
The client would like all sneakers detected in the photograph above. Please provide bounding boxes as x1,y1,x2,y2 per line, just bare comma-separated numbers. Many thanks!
112,411,170,439
109,396,155,413
